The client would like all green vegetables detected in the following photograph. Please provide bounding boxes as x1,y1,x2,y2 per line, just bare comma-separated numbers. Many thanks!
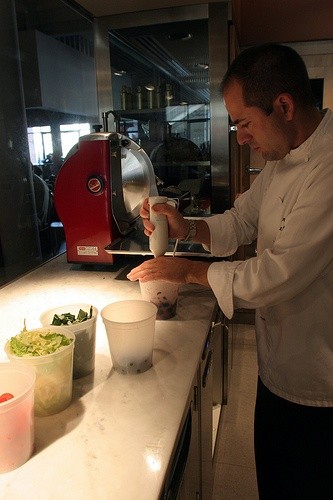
51,306,94,378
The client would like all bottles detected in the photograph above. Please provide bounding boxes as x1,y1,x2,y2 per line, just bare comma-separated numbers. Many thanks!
149,196,169,258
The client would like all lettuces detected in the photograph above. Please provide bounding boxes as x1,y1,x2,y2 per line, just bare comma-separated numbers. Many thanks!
8,318,73,417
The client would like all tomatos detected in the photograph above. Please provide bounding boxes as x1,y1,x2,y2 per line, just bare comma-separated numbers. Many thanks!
0,393,13,404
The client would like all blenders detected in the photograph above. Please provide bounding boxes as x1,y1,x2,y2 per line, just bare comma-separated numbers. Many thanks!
54,131,214,271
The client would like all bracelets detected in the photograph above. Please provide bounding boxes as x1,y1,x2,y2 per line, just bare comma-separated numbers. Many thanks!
180,219,196,243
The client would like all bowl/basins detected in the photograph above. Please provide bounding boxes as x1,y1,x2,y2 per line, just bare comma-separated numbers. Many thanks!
139,276,179,322
101,299,158,375
0,361,37,474
39,303,98,379
5,326,75,417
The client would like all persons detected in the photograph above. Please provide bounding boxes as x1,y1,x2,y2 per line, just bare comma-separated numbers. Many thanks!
30,162,50,228
124,44,333,500
32,166,42,179
